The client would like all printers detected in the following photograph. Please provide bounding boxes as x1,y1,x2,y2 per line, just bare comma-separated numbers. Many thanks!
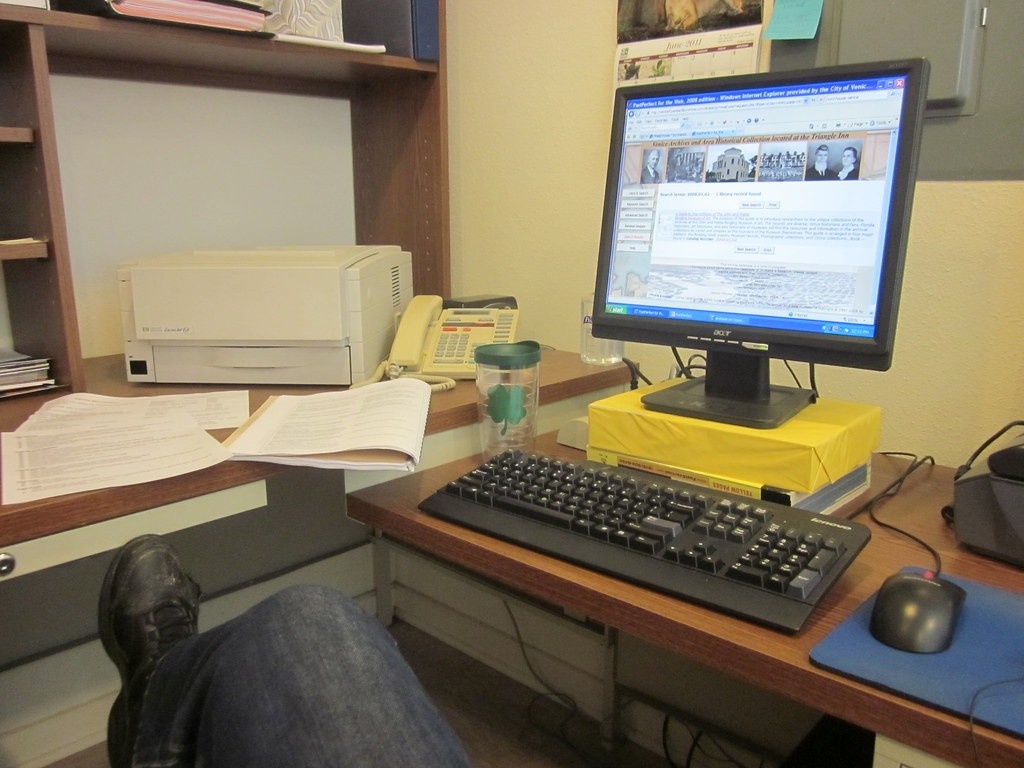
117,246,415,385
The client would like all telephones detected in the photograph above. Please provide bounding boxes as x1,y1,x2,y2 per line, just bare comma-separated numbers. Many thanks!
383,293,520,380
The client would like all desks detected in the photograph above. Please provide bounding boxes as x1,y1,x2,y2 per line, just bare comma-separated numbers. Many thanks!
346,431,1024,768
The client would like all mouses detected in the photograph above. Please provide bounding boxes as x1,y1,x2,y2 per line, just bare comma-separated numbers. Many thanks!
869,570,966,654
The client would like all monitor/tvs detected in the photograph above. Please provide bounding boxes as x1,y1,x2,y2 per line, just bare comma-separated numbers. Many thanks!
590,56,932,429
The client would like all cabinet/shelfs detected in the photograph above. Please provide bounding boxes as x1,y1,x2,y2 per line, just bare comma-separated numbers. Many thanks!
0,0,641,768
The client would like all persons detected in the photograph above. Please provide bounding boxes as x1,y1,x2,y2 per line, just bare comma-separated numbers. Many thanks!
641,150,661,185
805,145,861,181
99,533,474,768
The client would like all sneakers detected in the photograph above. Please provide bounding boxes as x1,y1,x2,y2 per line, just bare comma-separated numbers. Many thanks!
99,534,200,768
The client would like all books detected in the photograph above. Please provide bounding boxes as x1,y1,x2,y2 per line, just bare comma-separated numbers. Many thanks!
221,378,433,470
56,0,386,54
0,355,59,398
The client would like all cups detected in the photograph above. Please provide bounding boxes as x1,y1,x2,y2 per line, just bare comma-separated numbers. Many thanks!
473,340,541,464
580,292,623,366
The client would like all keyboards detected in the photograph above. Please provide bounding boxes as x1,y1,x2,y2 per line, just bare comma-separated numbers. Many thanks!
415,447,872,637
